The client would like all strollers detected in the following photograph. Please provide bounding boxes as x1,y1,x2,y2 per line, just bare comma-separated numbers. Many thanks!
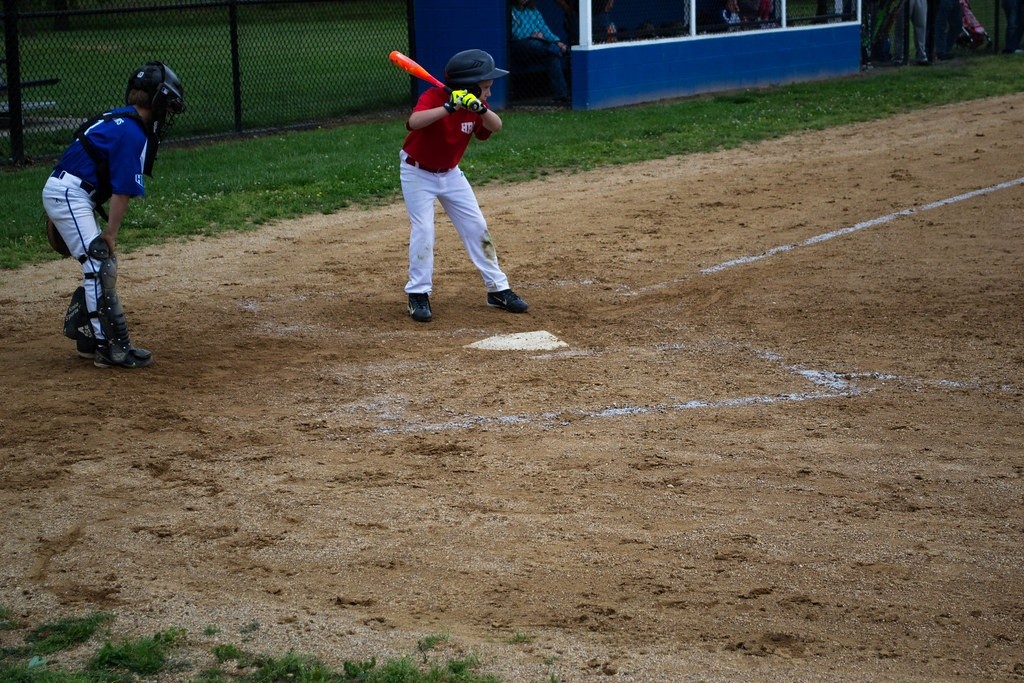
960,0,994,53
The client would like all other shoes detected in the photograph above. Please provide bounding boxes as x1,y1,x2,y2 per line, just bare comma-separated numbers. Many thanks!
893,59,903,64
918,61,931,66
1002,49,1015,55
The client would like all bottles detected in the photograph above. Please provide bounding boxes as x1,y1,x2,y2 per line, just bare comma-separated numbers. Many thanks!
607,22,617,43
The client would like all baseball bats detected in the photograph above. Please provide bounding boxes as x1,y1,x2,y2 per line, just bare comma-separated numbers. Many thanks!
388,50,479,112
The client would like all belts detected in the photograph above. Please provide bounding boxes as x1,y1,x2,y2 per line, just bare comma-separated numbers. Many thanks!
406,156,449,174
51,169,94,194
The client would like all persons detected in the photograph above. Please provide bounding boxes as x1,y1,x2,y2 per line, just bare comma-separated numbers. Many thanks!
509,0,1024,111
399,48,530,322
42,61,184,370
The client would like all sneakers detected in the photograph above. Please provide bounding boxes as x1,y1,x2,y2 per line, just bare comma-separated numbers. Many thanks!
487,289,528,313
78,340,97,358
93,346,153,369
407,292,432,322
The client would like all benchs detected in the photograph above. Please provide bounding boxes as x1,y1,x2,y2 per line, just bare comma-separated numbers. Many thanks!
511,54,569,75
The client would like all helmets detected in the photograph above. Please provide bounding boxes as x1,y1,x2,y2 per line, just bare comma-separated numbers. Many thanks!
446,49,510,98
125,61,184,150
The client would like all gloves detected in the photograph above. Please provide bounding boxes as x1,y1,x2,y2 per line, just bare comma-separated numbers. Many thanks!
444,89,468,113
460,93,487,114
